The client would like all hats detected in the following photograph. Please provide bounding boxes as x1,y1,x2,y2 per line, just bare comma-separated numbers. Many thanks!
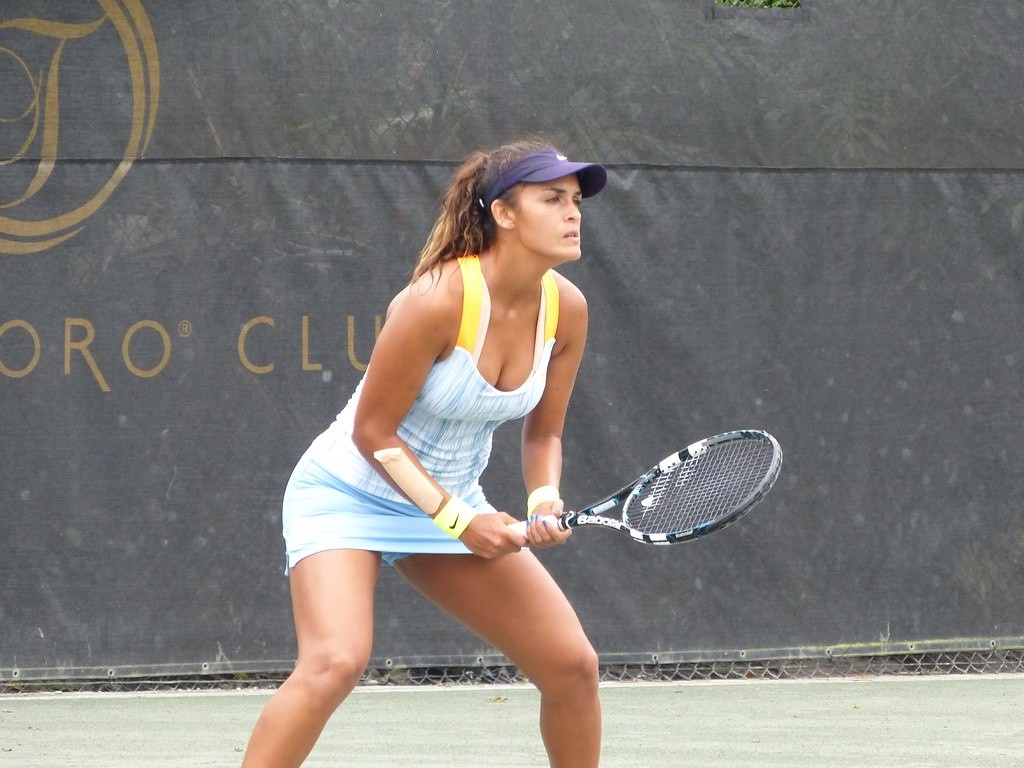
477,149,608,209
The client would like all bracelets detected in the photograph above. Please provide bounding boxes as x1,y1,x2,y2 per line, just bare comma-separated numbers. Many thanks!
432,495,477,541
527,485,560,522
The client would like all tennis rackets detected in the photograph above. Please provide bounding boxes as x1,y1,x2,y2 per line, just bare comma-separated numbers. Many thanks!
505,428,781,545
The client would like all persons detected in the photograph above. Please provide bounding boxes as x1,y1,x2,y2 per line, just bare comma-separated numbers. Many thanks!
234,140,612,768
